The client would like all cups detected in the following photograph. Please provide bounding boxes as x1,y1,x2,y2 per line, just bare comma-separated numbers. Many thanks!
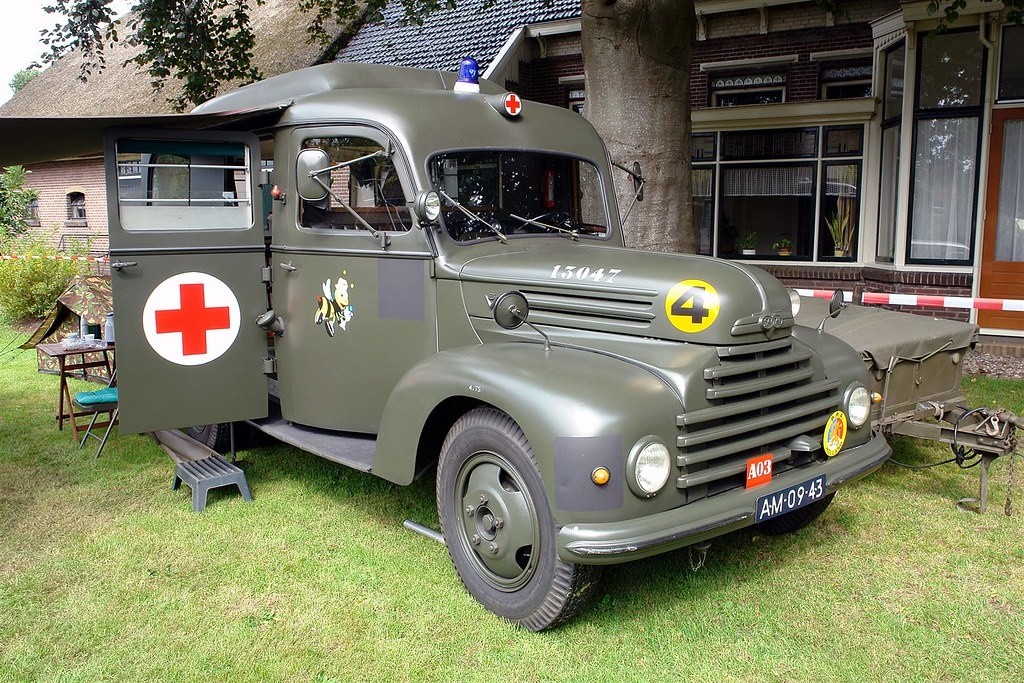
62,334,107,350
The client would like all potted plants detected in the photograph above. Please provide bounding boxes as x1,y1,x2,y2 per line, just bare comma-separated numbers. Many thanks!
773,239,794,257
825,212,854,257
739,230,760,255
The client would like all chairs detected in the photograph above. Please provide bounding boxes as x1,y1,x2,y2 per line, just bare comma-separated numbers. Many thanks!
72,366,165,461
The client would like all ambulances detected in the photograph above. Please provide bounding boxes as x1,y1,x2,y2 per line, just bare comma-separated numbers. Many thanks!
105,63,894,633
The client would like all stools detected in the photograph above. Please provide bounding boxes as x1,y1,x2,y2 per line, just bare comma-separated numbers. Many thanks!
172,452,254,513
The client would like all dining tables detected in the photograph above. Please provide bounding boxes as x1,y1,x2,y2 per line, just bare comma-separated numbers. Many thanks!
37,337,116,442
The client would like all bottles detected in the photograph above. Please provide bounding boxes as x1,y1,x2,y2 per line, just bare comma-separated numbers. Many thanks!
81,313,88,341
88,323,101,339
105,312,115,345
